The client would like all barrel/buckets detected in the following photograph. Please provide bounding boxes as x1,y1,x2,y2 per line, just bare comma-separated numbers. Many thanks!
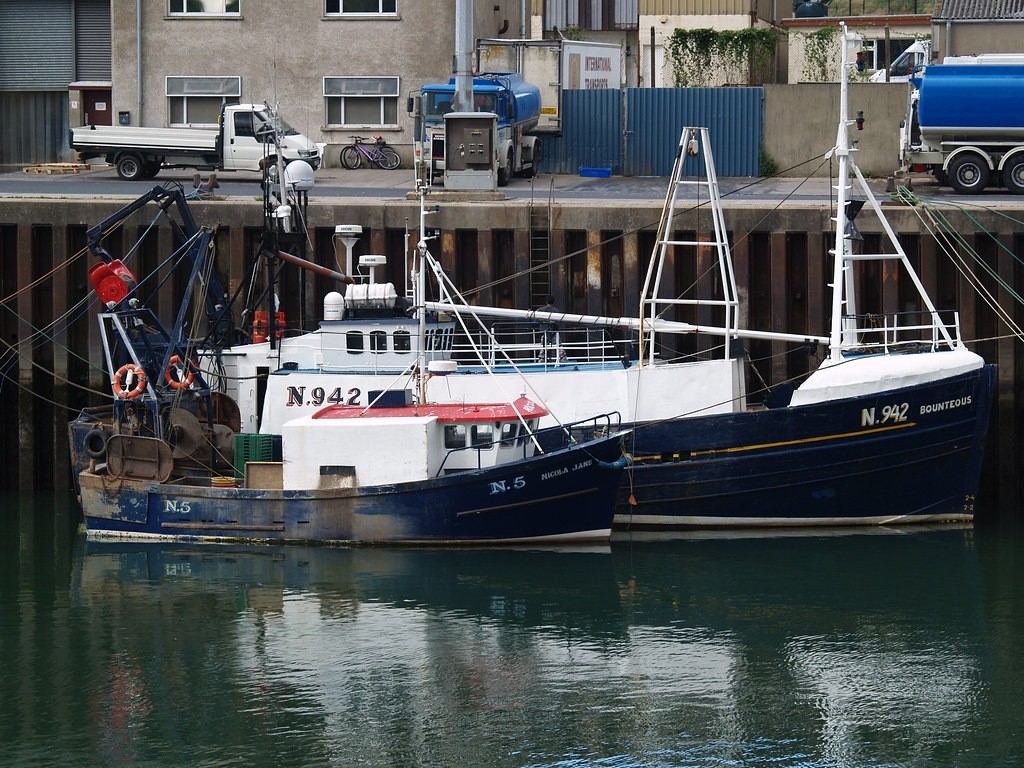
211,477,236,488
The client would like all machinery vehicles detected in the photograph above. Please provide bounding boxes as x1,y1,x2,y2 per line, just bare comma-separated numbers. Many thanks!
406,71,542,186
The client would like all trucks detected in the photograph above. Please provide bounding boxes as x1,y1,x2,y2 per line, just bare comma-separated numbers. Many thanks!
68,100,322,183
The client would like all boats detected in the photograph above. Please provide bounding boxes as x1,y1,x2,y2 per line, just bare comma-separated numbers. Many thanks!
0,59,634,550
374,17,999,530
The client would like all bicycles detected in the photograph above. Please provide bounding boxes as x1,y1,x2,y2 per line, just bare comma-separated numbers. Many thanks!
340,135,401,170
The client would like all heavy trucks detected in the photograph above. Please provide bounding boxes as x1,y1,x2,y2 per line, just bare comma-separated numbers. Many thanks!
897,48,1023,196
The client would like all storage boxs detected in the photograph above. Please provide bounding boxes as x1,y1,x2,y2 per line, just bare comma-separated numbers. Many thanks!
579,167,611,178
232,434,273,485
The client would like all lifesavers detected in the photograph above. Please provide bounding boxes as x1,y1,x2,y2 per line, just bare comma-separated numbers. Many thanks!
165,355,195,390
83,429,111,459
113,364,146,400
